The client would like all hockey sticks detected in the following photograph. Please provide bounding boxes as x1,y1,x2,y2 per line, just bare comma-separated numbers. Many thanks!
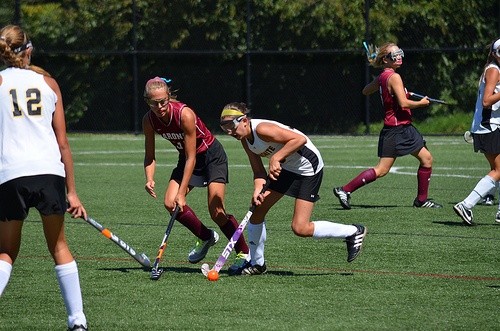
63,202,152,269
409,91,450,106
148,202,180,280
201,161,283,278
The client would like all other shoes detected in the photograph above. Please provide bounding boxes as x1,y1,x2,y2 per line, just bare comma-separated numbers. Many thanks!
67,318,89,330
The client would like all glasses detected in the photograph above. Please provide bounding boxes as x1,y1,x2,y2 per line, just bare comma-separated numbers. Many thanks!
384,49,404,61
145,96,170,108
492,49,500,58
219,114,246,133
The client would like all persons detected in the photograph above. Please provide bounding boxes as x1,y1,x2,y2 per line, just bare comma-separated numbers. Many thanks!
143,77,252,276
333,42,443,209
453,35,500,225
220,103,367,275
0,24,88,331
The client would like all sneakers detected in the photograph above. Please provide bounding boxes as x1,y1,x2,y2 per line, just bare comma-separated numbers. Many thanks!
482,195,499,206
235,258,268,275
229,248,252,271
494,208,500,223
188,228,219,263
453,201,478,226
332,187,352,209
413,197,443,208
343,223,367,262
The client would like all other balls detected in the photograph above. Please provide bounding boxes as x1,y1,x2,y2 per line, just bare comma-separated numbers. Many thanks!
208,271,219,280
